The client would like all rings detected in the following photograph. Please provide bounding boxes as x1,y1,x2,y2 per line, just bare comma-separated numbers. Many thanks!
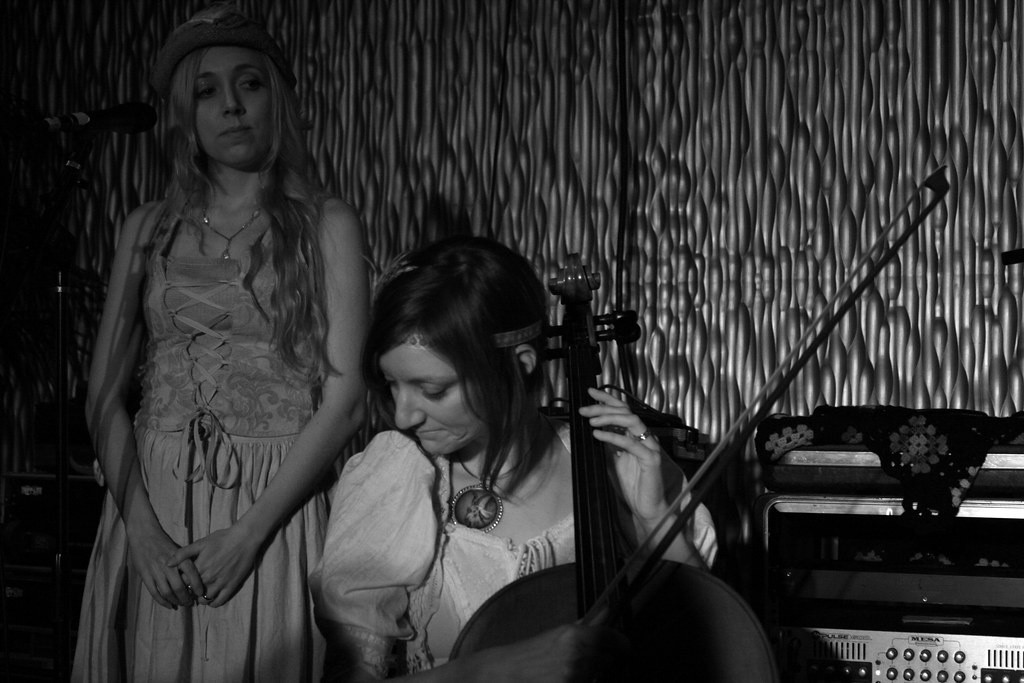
202,594,213,602
633,429,651,441
187,585,194,592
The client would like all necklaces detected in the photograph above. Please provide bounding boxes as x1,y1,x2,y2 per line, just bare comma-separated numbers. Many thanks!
195,202,264,265
446,455,518,532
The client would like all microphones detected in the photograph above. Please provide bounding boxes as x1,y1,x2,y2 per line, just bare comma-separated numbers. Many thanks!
44,101,157,133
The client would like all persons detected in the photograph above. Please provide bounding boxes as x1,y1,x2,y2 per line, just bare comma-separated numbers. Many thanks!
69,3,372,683
310,235,719,683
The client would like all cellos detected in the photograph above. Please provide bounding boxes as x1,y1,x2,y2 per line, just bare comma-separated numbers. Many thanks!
443,246,781,683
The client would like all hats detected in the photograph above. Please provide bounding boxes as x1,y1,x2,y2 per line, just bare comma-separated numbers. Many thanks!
149,7,297,98
